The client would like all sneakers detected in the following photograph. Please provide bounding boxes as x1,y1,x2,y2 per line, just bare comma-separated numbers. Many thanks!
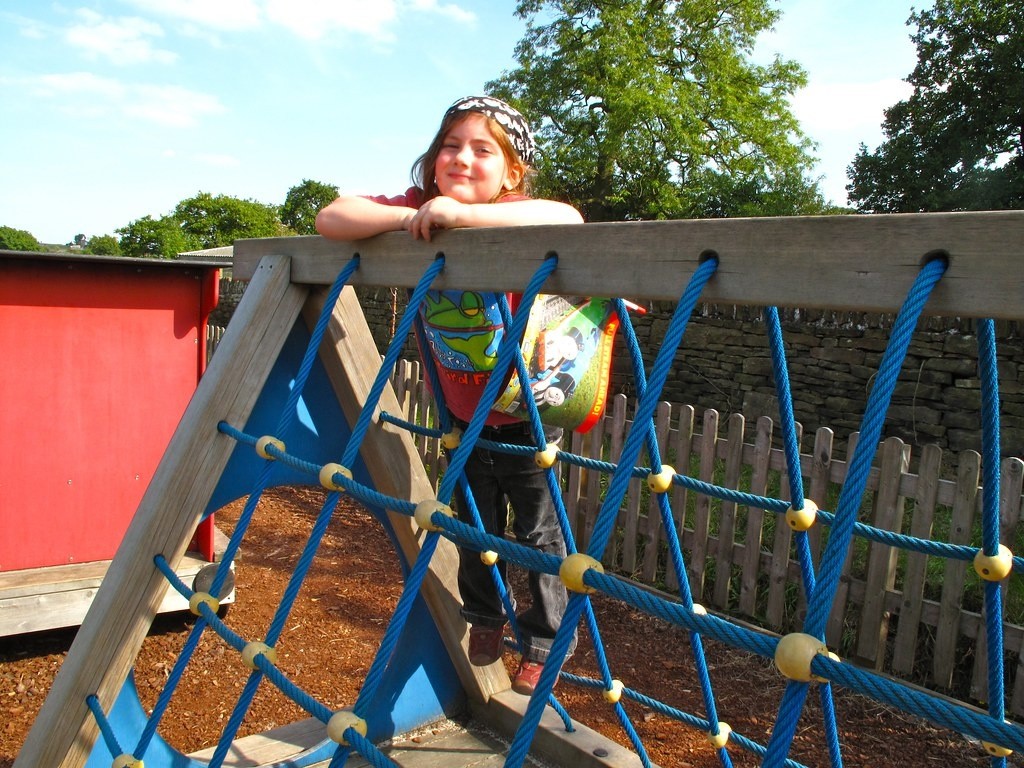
511,656,559,695
468,623,505,666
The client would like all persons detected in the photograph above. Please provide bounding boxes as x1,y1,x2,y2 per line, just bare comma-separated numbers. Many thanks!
313,92,589,699
